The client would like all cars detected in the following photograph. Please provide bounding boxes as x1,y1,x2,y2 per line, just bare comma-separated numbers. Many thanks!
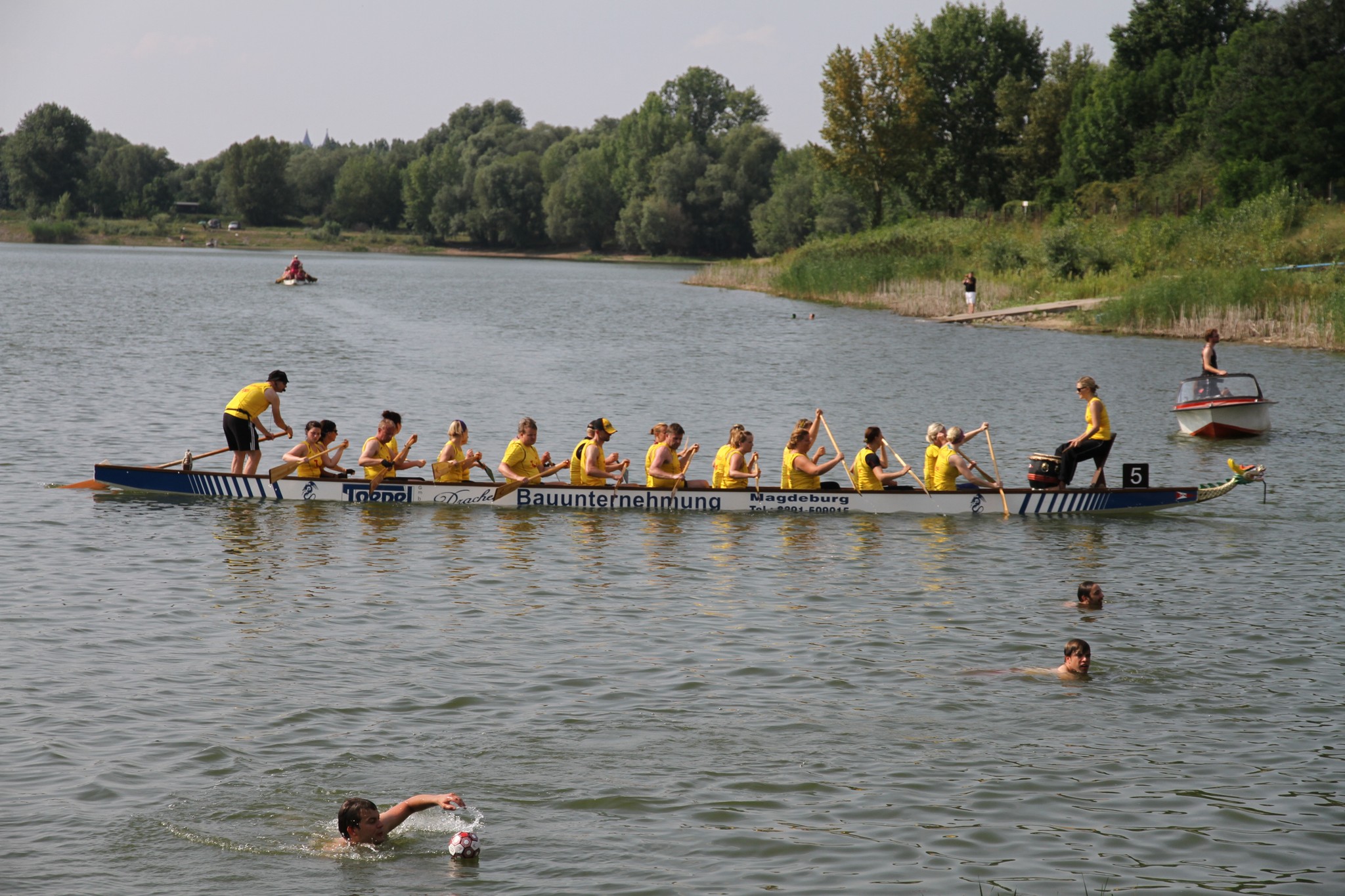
228,221,243,229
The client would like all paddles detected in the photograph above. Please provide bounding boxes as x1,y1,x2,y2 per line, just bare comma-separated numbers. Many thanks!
683,436,689,450
881,438,932,499
369,435,417,497
269,440,348,484
670,448,696,504
616,460,628,484
985,429,1009,513
493,462,567,501
548,459,560,482
754,455,761,503
820,415,863,497
613,463,627,501
432,452,481,479
60,428,292,489
958,450,997,483
467,450,495,482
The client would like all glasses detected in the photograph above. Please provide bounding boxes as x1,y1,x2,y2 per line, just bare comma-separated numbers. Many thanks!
1077,386,1086,392
1215,334,1219,338
333,430,337,433
282,382,287,384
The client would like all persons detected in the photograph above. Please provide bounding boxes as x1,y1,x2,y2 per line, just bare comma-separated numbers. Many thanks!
962,271,977,314
1058,638,1092,680
792,313,796,318
646,422,710,489
809,314,815,318
223,369,293,475
712,423,761,491
181,234,184,244
1197,328,1227,398
436,419,483,482
329,793,468,846
497,417,570,487
924,421,1003,491
781,408,844,491
1044,376,1111,491
1065,581,1105,607
570,417,639,487
275,254,318,284
358,409,427,481
281,419,355,479
849,426,911,491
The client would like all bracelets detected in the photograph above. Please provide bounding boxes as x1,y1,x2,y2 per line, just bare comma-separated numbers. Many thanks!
404,443,411,449
284,426,289,431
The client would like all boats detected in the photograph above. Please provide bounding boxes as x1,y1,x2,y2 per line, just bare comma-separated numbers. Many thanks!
96,460,1268,515
1170,374,1278,440
282,278,308,286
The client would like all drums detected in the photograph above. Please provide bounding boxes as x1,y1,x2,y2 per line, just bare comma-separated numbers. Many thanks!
1028,453,1064,489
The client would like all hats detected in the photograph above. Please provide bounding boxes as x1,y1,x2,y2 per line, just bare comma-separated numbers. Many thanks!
594,418,617,434
587,420,596,429
294,255,298,257
266,370,289,383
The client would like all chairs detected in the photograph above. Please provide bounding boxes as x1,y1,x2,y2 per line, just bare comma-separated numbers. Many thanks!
1076,432,1117,488
